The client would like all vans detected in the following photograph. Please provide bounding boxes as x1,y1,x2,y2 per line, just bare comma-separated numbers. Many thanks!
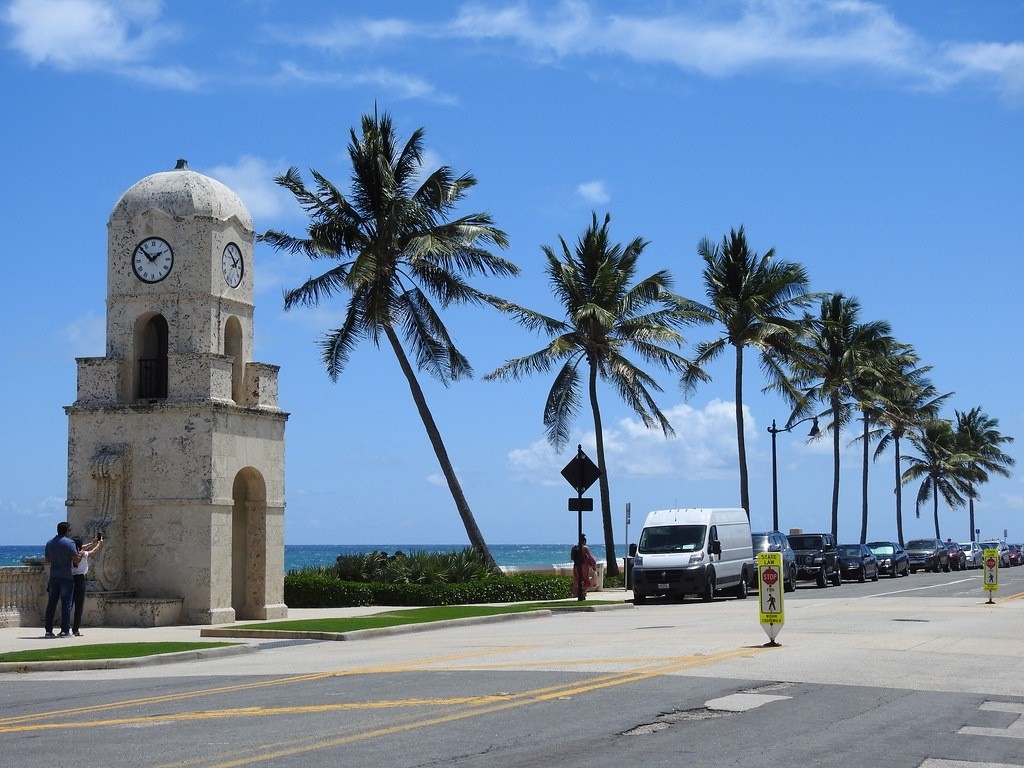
629,508,754,604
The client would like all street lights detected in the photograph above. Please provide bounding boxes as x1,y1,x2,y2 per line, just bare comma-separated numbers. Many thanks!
767,416,821,532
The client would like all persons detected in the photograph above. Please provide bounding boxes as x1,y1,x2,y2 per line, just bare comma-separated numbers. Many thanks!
57,536,103,635
571,534,597,599
44,522,84,637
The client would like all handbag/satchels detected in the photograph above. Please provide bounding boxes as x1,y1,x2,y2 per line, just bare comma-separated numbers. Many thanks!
590,571,599,588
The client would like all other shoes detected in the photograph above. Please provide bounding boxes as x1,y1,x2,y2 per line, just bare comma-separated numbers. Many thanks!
60,632,70,638
74,631,83,636
45,632,55,637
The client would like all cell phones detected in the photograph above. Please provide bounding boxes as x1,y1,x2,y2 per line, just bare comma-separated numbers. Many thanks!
98,533,101,541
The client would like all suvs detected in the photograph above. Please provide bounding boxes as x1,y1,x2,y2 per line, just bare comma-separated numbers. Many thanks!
976,538,1011,568
784,533,842,587
750,530,799,591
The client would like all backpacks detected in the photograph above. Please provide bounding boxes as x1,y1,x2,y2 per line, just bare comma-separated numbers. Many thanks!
572,545,582,562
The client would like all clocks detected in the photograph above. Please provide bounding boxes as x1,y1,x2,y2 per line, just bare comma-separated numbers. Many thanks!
221,242,244,288
131,237,174,283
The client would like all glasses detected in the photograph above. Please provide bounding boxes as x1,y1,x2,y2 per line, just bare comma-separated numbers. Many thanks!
67,529,72,531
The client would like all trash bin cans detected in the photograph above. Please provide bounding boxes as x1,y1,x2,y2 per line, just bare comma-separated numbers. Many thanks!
587,562,604,592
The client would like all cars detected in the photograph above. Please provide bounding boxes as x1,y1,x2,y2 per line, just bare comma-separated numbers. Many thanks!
834,544,880,583
1007,544,1024,566
942,541,967,570
957,541,984,569
978,544,994,555
903,539,951,573
864,541,911,578
